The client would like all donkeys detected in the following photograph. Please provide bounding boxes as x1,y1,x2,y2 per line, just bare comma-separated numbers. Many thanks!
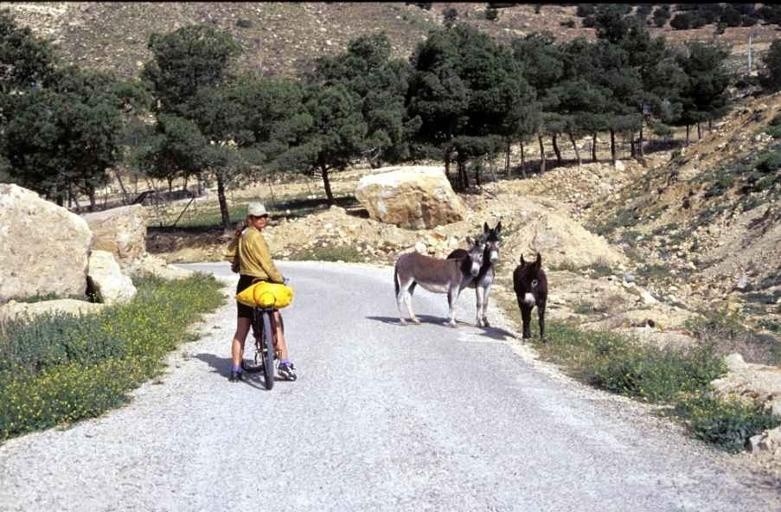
393,236,490,328
513,253,548,346
446,222,502,327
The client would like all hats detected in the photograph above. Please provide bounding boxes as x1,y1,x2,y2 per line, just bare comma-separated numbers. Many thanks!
247,201,271,217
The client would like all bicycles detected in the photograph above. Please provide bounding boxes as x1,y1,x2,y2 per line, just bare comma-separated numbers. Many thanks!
226,257,290,391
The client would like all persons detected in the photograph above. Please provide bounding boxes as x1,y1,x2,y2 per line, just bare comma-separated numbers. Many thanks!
228,202,297,382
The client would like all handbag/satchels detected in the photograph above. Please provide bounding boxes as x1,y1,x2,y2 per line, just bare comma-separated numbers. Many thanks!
234,281,294,310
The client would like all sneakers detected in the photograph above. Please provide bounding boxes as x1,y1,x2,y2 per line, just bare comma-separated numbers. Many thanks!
277,363,297,381
229,369,243,383
